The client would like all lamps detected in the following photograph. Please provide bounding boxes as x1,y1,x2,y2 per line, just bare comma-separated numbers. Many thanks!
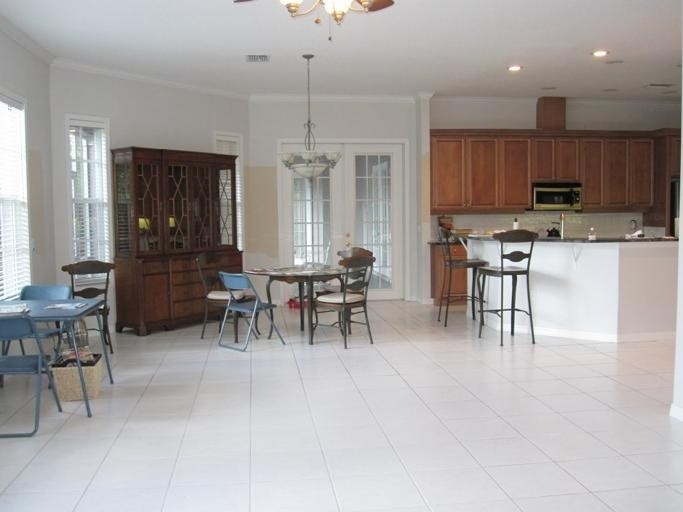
275,52,343,185
277,0,395,41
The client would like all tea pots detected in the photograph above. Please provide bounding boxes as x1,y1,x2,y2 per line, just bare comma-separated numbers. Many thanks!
544,222,560,237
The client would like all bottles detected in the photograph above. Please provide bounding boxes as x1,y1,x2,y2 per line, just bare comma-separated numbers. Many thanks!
587,227,597,240
512,218,518,230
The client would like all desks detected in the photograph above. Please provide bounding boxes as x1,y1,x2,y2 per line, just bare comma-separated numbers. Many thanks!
0,296,119,419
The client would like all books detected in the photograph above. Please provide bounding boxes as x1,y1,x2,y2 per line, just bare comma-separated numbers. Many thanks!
0,302,87,315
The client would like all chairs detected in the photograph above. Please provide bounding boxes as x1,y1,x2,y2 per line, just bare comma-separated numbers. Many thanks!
2,282,73,389
53,260,119,353
433,224,491,331
475,227,539,348
192,243,379,358
0,313,69,437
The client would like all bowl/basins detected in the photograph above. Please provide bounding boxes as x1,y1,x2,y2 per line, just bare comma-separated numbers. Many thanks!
453,228,472,237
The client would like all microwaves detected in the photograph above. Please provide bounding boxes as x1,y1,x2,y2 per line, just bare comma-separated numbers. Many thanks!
531,183,582,212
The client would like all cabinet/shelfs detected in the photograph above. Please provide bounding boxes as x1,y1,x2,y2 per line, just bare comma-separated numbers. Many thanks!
532,130,579,182
429,242,467,308
496,129,532,213
642,129,680,237
108,145,245,342
576,129,603,210
600,131,652,212
428,128,499,214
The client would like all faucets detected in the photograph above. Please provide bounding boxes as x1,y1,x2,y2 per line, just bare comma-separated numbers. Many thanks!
631,219,637,231
